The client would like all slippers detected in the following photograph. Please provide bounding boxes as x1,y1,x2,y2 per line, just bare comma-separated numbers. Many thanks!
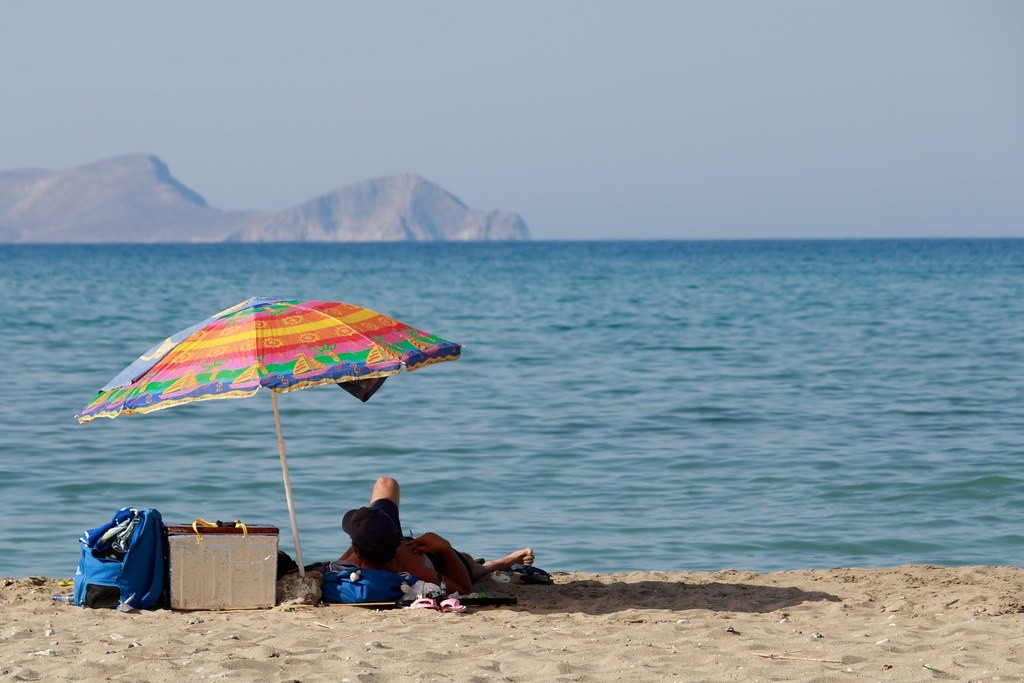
410,597,440,610
439,597,468,613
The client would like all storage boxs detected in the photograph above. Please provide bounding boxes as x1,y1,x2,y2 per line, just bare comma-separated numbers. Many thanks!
165,522,279,611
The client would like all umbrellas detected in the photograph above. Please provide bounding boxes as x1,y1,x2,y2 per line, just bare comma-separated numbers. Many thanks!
78,297,463,577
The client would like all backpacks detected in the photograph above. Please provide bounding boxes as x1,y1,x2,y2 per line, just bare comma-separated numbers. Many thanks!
72,506,166,613
321,560,420,604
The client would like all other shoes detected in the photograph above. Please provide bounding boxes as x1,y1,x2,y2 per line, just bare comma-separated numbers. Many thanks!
511,563,547,576
520,575,552,585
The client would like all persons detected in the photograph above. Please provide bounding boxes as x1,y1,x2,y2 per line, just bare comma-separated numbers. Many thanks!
338,476,534,595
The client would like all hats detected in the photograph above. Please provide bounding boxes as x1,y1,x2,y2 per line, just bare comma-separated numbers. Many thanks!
342,505,402,556
